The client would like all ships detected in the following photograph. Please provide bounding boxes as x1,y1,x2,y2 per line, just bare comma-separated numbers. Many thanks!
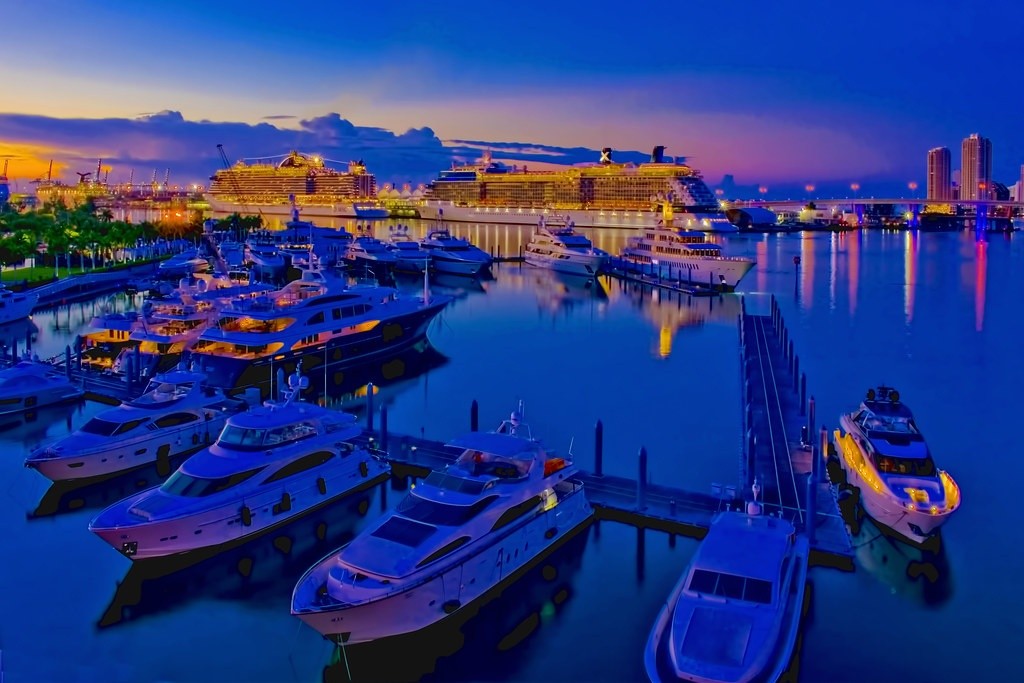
35,178,115,208
202,149,390,218
410,146,740,232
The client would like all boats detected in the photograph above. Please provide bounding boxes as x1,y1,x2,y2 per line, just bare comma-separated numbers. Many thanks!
289,411,595,644
111,294,226,379
833,385,961,544
645,476,810,683
24,361,246,481
245,231,286,279
524,210,609,276
609,226,758,288
421,209,494,275
80,312,141,354
181,258,455,394
386,225,431,272
159,251,210,273
0,362,85,424
90,358,391,560
270,195,354,265
340,224,397,271
0,284,39,323
